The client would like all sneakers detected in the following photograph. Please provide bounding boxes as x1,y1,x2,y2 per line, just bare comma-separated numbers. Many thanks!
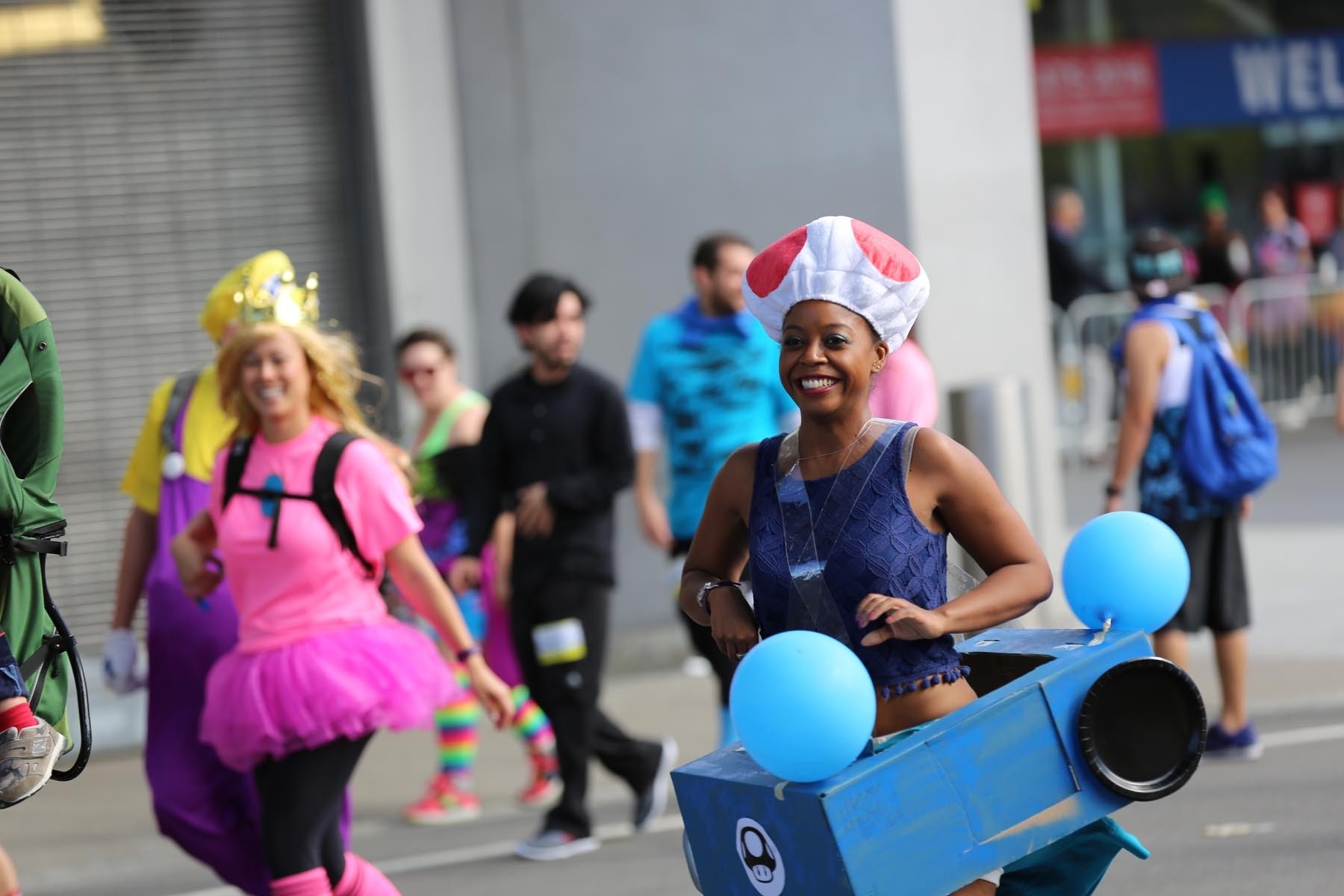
0,715,67,809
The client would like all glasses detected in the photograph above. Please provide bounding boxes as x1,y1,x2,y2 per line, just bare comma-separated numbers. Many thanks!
398,354,450,382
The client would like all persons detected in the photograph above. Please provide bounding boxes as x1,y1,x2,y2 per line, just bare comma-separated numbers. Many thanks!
1048,182,1330,421
0,643,67,813
168,319,515,896
470,274,675,860
678,216,1056,896
99,249,427,896
871,334,938,427
630,234,801,557
1103,230,1261,761
390,328,562,825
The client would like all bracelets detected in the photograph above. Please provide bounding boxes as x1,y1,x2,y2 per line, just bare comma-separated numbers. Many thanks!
696,580,741,615
453,647,481,664
1105,485,1122,497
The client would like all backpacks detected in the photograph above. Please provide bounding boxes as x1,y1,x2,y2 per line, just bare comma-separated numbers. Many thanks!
1108,305,1282,505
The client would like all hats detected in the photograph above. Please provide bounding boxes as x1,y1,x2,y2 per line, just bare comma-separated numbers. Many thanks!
741,213,928,358
200,249,313,347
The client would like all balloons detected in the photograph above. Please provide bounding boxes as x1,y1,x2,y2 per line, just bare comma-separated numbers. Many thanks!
729,628,879,784
1062,510,1191,635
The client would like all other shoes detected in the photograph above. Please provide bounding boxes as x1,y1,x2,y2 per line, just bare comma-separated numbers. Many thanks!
633,740,677,831
520,753,565,810
406,778,480,825
1200,721,1262,758
517,821,600,860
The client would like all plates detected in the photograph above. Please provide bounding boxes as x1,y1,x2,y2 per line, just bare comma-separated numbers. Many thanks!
1079,656,1206,803
